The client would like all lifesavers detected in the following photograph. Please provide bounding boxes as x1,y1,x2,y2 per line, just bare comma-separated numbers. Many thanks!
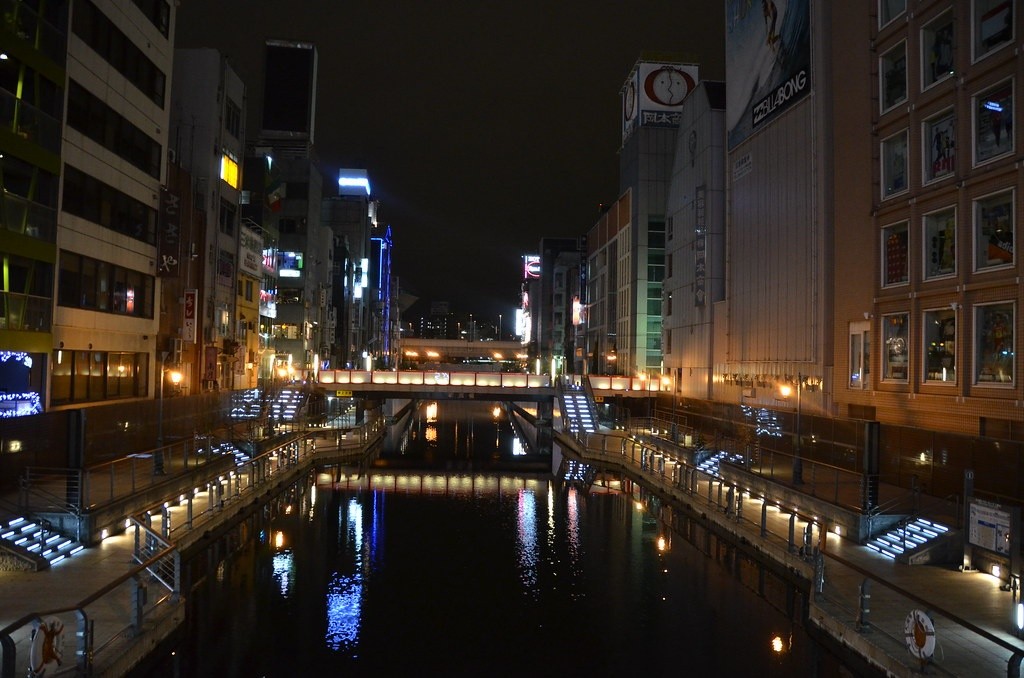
902,607,938,661
26,616,69,678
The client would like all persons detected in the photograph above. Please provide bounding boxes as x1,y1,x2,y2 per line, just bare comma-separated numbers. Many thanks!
990,314,1006,372
988,97,1012,148
759,0,783,52
931,126,954,170
